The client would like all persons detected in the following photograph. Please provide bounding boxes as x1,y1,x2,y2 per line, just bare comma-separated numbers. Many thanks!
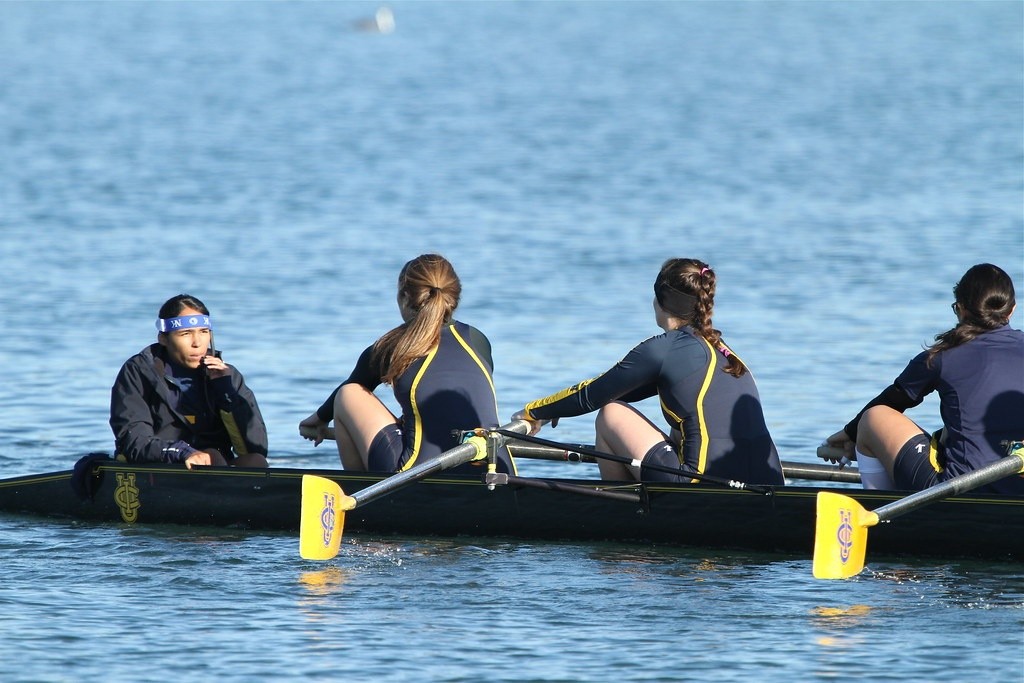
821,262,1024,494
109,295,269,469
299,254,518,476
511,258,785,486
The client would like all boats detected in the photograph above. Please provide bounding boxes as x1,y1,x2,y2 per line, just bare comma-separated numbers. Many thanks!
0,464,1024,567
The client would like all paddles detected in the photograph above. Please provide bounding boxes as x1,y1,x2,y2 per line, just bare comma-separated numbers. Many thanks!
299,418,550,562
506,437,861,484
812,447,1024,581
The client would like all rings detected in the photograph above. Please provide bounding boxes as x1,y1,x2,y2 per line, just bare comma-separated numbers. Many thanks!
841,456,852,467
822,440,829,447
